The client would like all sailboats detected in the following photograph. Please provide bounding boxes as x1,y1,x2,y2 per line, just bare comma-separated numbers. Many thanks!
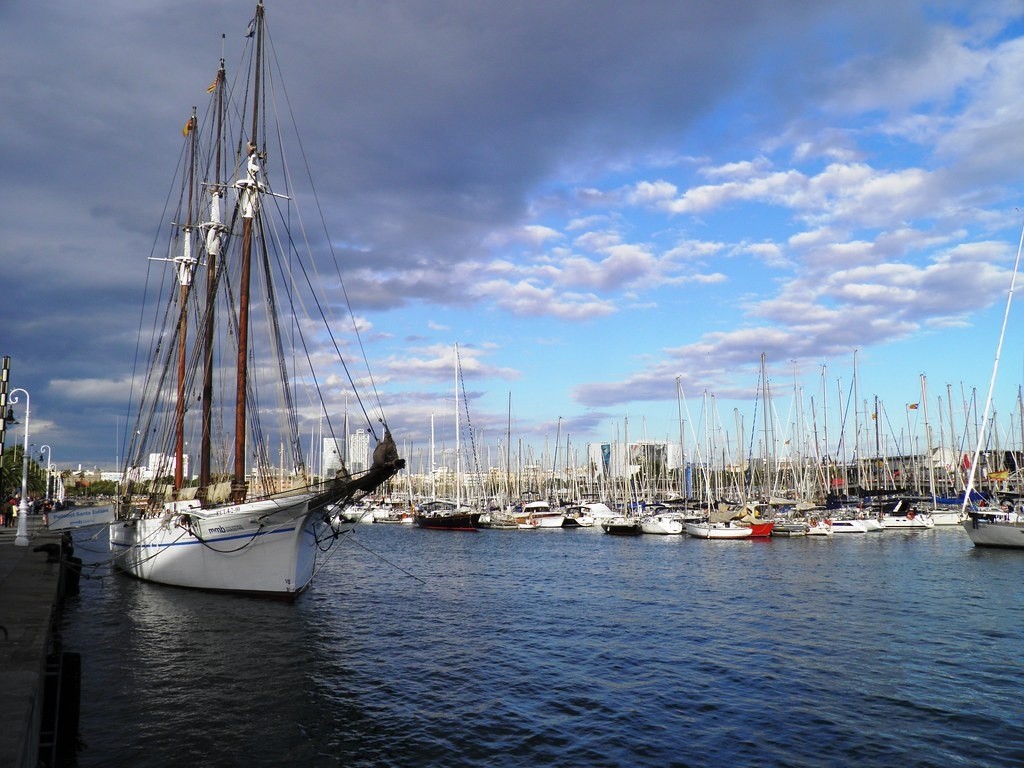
321,348,982,540
106,13,405,600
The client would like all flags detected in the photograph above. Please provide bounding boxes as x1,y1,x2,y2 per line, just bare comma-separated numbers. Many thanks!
871,413,876,421
909,404,918,410
206,69,225,93
183,119,198,137
785,439,791,445
988,470,1009,482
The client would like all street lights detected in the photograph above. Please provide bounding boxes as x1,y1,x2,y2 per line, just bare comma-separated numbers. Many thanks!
50,462,58,508
4,389,35,547
39,444,52,510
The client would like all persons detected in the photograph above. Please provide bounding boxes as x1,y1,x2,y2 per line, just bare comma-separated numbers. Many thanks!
411,498,423,515
755,509,761,517
0,496,61,528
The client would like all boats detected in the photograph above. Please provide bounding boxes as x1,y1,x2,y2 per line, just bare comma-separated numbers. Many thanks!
963,509,1023,549
